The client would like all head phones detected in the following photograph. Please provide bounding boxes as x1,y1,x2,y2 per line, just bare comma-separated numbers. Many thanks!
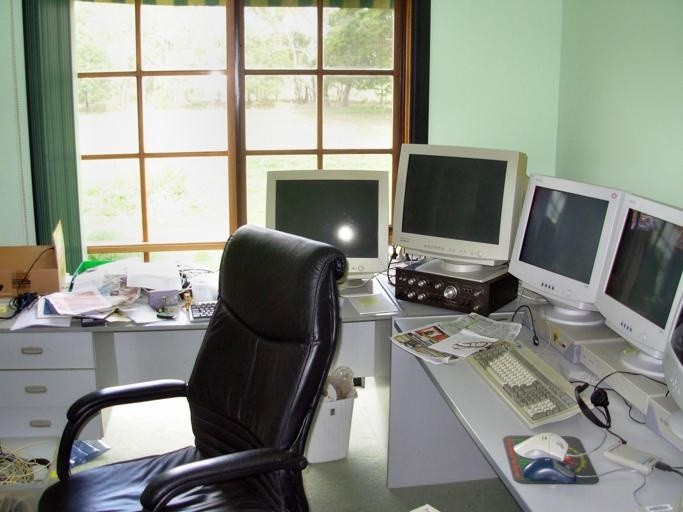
573,379,612,428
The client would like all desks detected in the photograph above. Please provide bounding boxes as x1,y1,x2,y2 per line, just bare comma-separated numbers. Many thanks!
372,264,552,378
0,253,402,449
387,309,683,510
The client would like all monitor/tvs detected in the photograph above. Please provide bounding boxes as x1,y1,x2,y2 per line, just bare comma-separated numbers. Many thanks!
507,174,625,327
266,170,389,296
595,192,683,377
664,295,683,411
392,143,528,285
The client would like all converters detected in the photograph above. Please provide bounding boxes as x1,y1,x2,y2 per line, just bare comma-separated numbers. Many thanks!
602,439,661,475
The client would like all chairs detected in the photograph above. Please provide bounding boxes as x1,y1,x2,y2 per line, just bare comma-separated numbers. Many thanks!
38,224,346,512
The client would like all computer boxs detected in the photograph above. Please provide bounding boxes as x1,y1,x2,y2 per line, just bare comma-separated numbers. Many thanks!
647,398,681,454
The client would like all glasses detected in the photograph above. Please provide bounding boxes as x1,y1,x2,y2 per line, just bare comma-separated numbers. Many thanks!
452,341,487,349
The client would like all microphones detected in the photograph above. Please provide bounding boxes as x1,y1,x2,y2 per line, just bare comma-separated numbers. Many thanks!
511,304,539,345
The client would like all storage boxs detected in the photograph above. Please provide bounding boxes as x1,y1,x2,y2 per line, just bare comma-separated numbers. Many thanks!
0,438,62,511
0,220,68,295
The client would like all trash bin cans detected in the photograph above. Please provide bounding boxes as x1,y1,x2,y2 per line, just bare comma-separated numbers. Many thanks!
303,375,358,463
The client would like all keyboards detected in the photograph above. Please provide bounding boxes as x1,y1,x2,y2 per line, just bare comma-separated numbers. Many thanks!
467,338,595,428
189,301,220,322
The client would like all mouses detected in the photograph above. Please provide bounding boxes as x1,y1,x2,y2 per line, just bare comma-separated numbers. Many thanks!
520,457,576,484
513,431,569,461
